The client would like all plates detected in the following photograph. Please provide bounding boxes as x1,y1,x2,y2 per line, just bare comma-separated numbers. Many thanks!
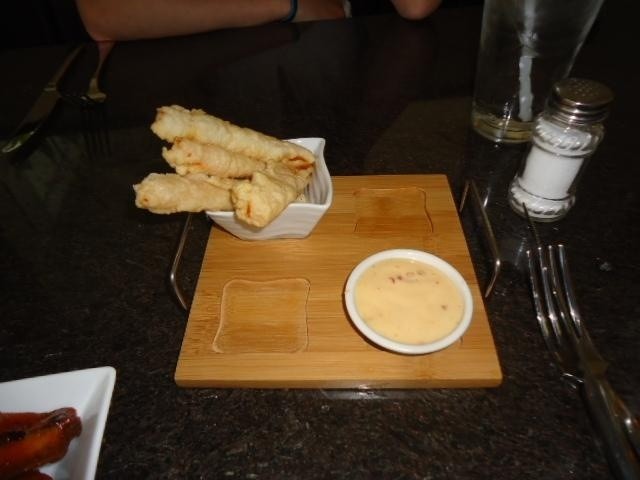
345,247,473,356
1,364,118,480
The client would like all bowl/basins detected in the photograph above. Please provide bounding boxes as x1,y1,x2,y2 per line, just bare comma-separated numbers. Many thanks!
205,136,332,242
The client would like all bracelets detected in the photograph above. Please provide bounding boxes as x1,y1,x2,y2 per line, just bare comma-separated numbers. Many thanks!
282,0,298,24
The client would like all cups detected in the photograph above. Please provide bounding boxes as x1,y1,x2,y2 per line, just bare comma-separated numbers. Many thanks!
469,0,614,146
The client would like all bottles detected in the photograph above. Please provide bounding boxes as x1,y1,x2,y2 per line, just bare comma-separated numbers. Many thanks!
508,77,607,222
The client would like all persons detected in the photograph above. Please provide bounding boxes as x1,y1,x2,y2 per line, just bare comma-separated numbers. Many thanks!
75,0,443,44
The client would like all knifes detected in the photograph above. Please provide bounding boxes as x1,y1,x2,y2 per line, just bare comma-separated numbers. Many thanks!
1,41,86,158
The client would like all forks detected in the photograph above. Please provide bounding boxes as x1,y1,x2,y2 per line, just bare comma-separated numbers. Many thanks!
521,243,640,480
79,38,118,159
